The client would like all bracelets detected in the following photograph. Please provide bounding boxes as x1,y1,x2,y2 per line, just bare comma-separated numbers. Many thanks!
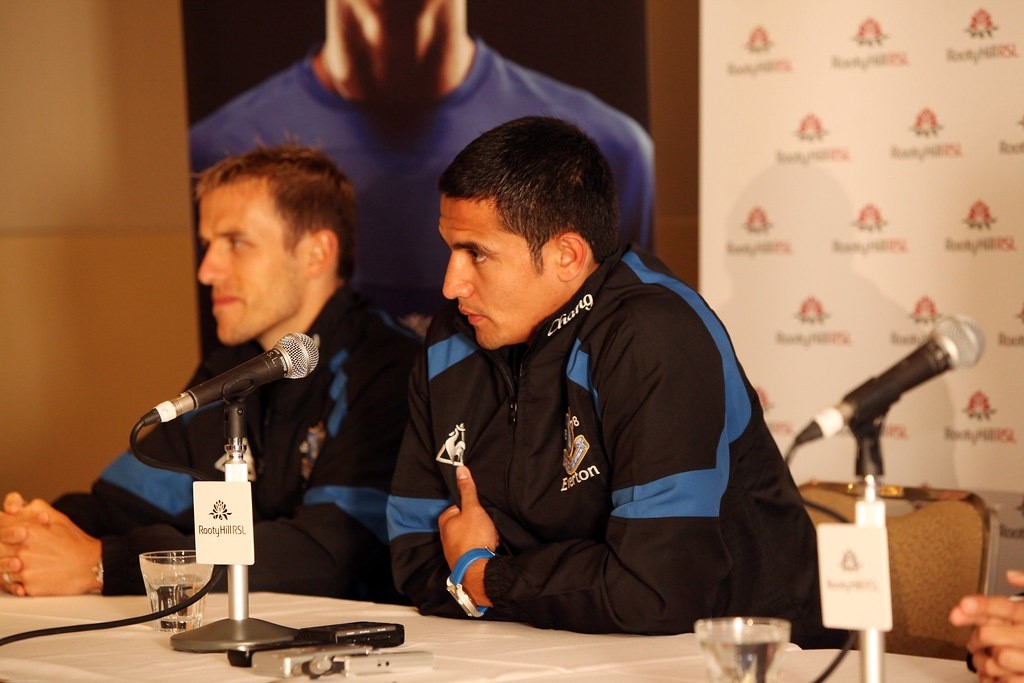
88,560,104,595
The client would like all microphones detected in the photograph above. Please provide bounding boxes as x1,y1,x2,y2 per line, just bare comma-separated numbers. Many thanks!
139,334,319,427
795,313,986,443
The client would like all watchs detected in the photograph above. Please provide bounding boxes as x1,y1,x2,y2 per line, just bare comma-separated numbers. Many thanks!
446,547,495,617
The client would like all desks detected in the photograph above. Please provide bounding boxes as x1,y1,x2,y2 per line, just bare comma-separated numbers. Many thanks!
0,591,981,683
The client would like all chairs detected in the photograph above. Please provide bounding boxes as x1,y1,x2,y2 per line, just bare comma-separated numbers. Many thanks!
797,480,1001,661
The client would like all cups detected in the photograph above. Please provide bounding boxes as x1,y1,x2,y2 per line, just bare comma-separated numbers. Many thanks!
694,617,792,683
139,550,214,634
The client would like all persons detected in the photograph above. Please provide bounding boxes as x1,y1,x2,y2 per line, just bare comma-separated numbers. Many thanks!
948,569,1024,683
187,0,655,360
0,145,428,604
385,116,850,651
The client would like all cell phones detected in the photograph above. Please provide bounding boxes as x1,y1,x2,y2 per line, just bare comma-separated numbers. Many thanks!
297,620,406,651
